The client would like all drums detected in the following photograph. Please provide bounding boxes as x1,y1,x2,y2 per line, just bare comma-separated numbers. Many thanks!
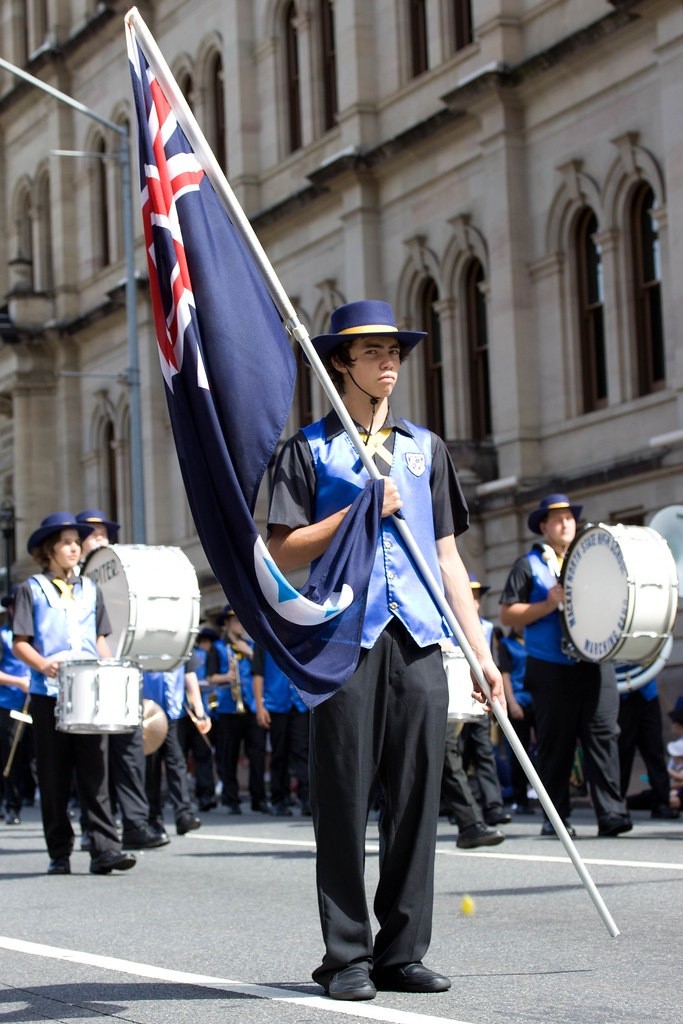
557,521,678,665
81,542,201,672
441,649,491,725
53,657,146,736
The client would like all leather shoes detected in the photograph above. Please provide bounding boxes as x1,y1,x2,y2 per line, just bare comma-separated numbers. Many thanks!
485,807,512,825
90,850,136,875
328,962,377,1000
598,812,633,835
370,962,452,992
122,801,240,849
542,817,575,835
457,824,504,848
49,855,70,872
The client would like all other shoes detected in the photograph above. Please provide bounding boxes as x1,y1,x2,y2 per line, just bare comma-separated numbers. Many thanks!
251,802,271,812
302,806,310,815
651,804,679,819
2,800,19,824
272,806,292,816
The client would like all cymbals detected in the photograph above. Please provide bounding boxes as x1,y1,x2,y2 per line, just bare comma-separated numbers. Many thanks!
182,702,212,750
142,698,169,756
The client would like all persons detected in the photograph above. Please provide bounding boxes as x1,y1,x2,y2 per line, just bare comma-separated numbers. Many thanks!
0,495,683,878
263,300,506,1000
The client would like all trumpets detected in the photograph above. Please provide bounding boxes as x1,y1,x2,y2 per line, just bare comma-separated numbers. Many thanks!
226,643,247,713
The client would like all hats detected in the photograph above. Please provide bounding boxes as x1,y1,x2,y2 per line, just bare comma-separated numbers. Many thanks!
27,513,94,555
528,493,582,536
1,585,18,606
215,605,236,626
76,510,120,536
200,628,220,642
304,301,426,364
468,572,490,596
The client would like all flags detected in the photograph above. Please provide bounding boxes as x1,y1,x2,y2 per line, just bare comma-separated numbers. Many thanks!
127,9,404,714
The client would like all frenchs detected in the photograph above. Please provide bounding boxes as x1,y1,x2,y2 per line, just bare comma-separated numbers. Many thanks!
616,503,683,693
